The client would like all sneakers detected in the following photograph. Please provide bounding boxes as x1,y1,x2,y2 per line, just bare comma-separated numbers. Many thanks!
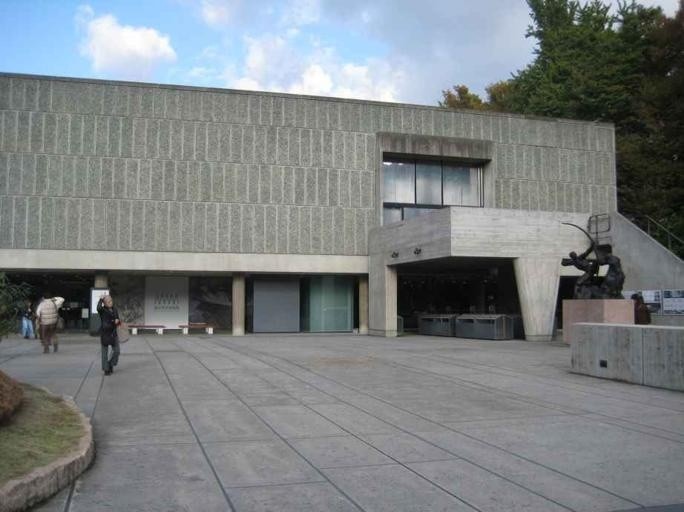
42,344,59,353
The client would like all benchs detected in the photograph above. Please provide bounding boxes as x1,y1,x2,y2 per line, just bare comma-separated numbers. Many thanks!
179,324,220,336
128,325,167,335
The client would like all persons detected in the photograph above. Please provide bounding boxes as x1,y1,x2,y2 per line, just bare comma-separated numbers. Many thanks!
34,292,65,354
558,240,601,298
97,293,122,377
20,300,35,339
594,242,625,297
631,292,651,325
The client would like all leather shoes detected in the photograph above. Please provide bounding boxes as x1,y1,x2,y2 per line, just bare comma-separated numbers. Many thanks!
103,360,113,376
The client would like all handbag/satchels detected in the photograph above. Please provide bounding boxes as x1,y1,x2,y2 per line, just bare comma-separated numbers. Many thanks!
55,316,66,331
116,321,130,344
